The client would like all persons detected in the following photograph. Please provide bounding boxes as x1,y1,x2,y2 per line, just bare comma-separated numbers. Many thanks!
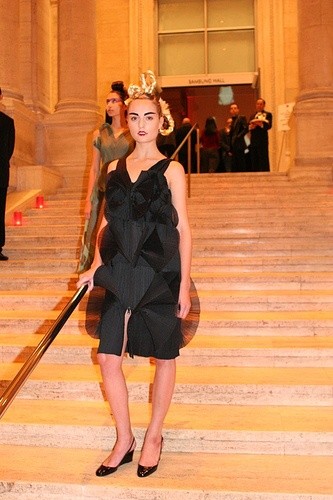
228,103,249,172
200,114,221,173
220,117,233,172
248,98,273,172
76,80,136,273
0,87,15,261
76,70,192,479
174,118,196,173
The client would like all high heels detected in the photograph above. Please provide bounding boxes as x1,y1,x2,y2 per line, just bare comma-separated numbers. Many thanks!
137,436,163,477
96,437,134,476
